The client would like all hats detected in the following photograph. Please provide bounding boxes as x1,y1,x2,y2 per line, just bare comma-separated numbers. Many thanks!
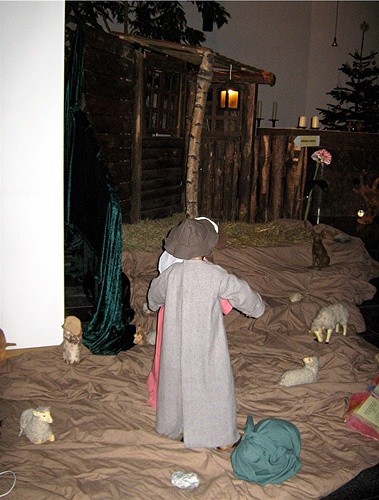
164,218,218,259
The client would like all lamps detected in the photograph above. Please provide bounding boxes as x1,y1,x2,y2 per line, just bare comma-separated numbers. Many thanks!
217,79,240,111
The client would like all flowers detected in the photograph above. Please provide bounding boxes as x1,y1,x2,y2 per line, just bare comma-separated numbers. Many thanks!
305,149,331,224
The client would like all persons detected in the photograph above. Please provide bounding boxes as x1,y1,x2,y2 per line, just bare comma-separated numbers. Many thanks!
147,217,265,451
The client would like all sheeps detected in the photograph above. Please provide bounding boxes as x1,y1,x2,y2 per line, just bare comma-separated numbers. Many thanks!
278,356,319,387
309,303,350,344
18,407,54,444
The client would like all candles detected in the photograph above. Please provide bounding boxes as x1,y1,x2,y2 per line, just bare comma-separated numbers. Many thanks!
312,116,320,128
298,116,306,127
272,101,278,120
257,101,262,118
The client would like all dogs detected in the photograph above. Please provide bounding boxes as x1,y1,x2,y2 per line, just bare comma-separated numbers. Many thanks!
309,229,330,268
